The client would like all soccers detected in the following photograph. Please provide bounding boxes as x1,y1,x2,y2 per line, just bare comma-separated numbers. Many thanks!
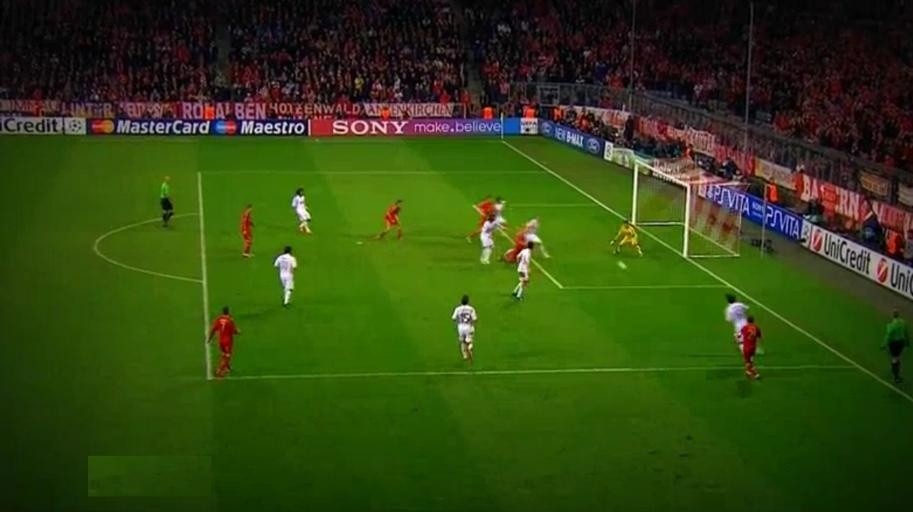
618,261,626,270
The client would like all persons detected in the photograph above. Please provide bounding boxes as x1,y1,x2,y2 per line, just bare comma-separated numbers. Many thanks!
879,307,909,383
736,314,761,380
373,198,404,241
291,187,312,234
206,306,241,380
802,200,824,226
884,225,905,260
239,202,255,258
608,220,643,259
723,292,766,355
763,177,779,205
159,174,174,227
451,294,477,360
464,196,552,301
273,246,297,309
901,228,913,266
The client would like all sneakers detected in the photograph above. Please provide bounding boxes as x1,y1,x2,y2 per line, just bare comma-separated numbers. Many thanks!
160,222,314,309
612,248,646,258
740,348,904,385
374,234,405,242
465,236,552,302
213,367,234,378
460,347,475,363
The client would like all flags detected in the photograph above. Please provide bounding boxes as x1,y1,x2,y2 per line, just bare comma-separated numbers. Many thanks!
856,186,884,250
